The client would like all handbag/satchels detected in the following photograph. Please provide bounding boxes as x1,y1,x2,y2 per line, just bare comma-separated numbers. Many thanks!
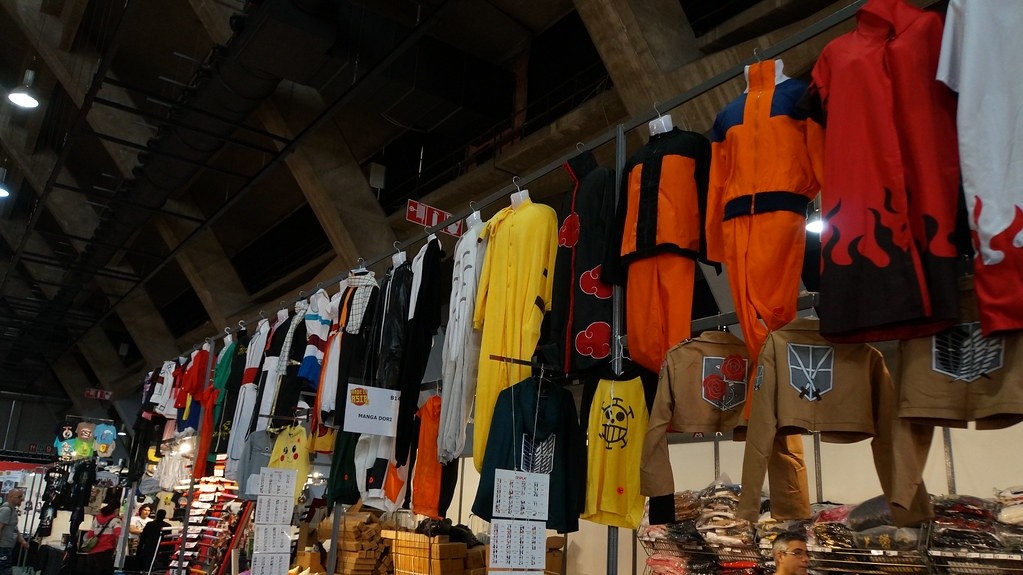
81,536,98,551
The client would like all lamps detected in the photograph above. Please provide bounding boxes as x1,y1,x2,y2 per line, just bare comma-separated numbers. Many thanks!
805,192,822,234
0,159,10,198
8,54,41,109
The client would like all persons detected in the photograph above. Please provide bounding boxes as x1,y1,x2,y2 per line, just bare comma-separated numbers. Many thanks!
772,532,814,575
88,500,121,575
0,489,29,575
127,503,172,572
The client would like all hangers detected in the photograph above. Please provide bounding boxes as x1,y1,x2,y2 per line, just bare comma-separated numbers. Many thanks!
803,292,818,318
432,378,441,398
607,337,633,364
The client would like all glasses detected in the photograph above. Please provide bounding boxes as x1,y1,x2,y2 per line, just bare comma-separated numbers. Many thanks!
783,551,811,559
13,495,22,499
